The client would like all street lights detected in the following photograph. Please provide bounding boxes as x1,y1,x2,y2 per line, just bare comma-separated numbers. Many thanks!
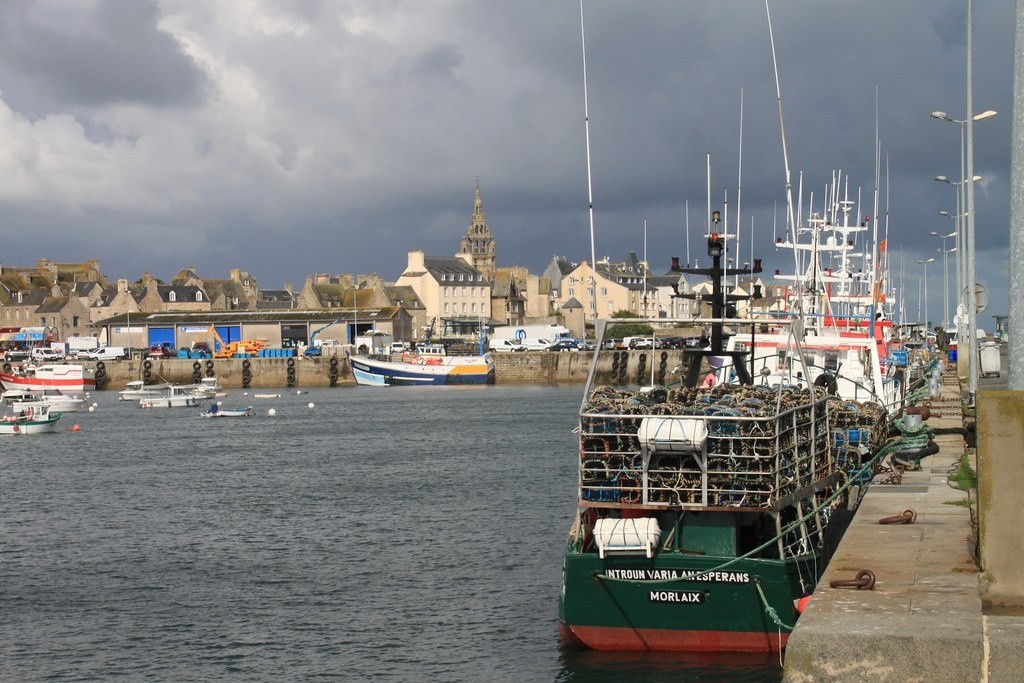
126,308,133,360
918,258,935,334
929,110,999,347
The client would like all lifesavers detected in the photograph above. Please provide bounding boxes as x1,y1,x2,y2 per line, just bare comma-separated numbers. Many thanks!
95,361,105,390
659,352,668,385
2,363,12,371
815,374,837,395
329,358,338,388
637,353,646,383
704,375,716,387
192,361,201,383
287,357,295,387
618,352,627,382
206,360,215,369
143,361,152,385
205,370,214,377
610,351,617,377
241,360,251,388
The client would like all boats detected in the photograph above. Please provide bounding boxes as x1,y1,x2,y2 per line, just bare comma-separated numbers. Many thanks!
192,374,227,399
0,364,97,393
200,404,254,418
254,393,282,399
0,388,87,438
117,380,160,399
140,386,201,408
347,290,497,389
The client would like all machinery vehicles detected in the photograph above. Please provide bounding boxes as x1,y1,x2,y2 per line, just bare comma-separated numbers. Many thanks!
191,325,269,359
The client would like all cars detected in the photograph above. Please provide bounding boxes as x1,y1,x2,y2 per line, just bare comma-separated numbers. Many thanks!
548,336,703,351
490,340,529,353
148,345,171,359
165,347,178,356
390,342,405,353
525,339,555,352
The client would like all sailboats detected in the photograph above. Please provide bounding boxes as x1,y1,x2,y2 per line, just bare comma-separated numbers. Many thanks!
555,89,921,655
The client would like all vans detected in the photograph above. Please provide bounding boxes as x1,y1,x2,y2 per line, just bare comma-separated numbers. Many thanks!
87,347,126,360
31,348,57,362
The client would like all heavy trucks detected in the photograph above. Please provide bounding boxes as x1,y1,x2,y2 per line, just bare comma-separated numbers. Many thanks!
494,324,570,342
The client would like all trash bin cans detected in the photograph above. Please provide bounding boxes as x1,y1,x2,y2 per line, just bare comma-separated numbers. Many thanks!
978,340,1002,378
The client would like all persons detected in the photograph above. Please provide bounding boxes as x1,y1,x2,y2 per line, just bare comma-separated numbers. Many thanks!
20,407,34,422
934,327,950,354
